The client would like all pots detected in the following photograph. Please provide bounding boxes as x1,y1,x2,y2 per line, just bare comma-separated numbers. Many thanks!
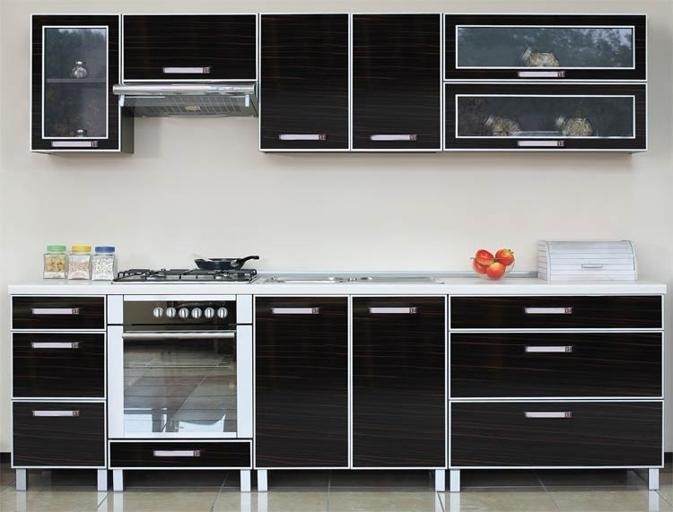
194,255,258,270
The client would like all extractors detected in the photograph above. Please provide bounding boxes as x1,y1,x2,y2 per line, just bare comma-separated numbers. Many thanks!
112,80,255,118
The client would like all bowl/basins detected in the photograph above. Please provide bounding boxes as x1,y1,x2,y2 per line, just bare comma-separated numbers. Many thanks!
472,256,515,282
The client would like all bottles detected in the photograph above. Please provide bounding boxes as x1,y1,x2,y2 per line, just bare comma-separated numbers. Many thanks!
69,246,92,280
72,61,88,79
485,113,521,136
44,246,68,279
522,46,560,67
75,130,88,137
92,246,118,281
555,113,598,136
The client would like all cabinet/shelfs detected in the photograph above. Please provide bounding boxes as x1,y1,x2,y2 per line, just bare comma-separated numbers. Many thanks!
30,15,134,154
442,13,649,152
107,294,252,493
113,15,258,82
253,294,448,492
261,14,442,150
10,296,106,492
448,296,664,492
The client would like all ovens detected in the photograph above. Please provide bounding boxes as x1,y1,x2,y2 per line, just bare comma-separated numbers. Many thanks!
106,295,254,440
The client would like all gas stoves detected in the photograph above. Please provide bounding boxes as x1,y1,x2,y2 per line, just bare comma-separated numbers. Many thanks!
109,267,257,285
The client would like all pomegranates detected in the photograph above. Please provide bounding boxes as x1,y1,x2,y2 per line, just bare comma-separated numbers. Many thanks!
473,249,513,279
